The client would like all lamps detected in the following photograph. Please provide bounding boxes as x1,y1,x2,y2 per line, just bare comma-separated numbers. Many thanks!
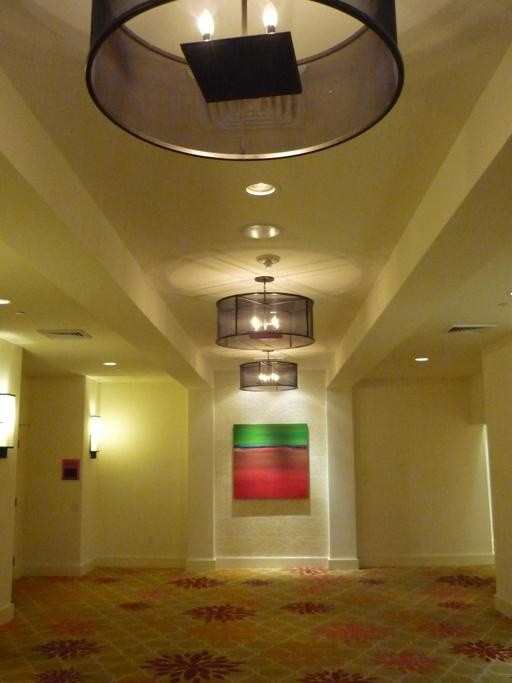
239,349,299,394
0,394,19,458
83,1,405,162
91,415,102,459
216,275,315,351
